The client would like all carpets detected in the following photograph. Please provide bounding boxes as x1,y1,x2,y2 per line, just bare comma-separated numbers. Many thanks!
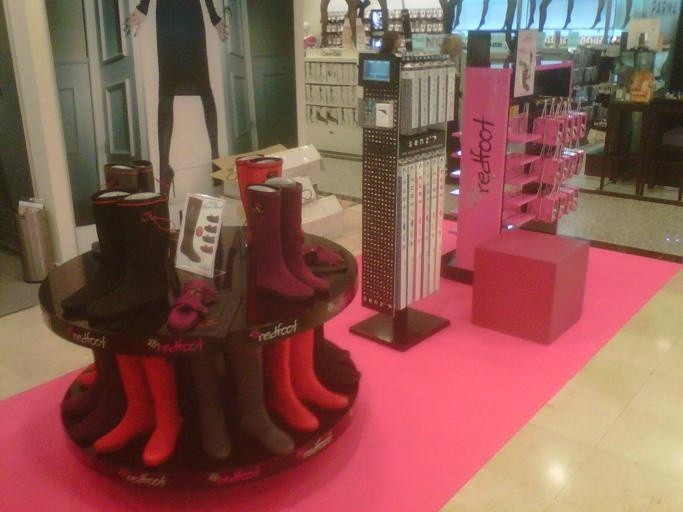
0,243,43,320
1,207,681,511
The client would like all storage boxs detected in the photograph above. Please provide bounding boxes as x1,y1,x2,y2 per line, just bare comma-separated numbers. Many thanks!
301,182,362,255
212,142,328,200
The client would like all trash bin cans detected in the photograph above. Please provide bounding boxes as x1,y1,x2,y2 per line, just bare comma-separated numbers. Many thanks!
12,204,55,283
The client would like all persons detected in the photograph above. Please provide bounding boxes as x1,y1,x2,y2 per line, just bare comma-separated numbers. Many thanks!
124,1,230,194
378,29,402,55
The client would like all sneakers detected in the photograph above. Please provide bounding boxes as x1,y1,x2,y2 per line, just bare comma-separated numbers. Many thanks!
204,224,218,235
200,245,214,255
206,214,220,224
202,234,215,245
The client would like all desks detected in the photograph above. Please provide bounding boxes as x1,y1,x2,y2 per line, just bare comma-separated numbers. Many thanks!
600,99,683,202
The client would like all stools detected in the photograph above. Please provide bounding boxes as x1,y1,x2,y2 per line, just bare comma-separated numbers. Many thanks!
472,228,588,345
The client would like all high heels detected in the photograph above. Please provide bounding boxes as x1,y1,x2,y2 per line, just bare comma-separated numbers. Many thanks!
158,168,175,204
211,160,222,188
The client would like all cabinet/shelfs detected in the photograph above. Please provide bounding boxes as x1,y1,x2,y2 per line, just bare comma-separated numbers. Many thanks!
306,47,363,203
39,227,359,488
440,29,588,285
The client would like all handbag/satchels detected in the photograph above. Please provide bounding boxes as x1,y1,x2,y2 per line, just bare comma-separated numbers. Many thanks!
287,175,316,205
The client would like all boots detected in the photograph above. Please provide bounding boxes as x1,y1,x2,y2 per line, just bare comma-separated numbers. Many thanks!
247,156,306,244
103,161,137,195
71,348,128,443
138,352,183,468
243,181,317,302
128,159,154,193
235,153,266,245
61,348,105,419
92,353,156,454
60,189,138,317
87,193,181,326
262,175,332,297
263,337,323,434
290,329,349,412
192,353,232,464
179,196,202,264
230,349,297,459
